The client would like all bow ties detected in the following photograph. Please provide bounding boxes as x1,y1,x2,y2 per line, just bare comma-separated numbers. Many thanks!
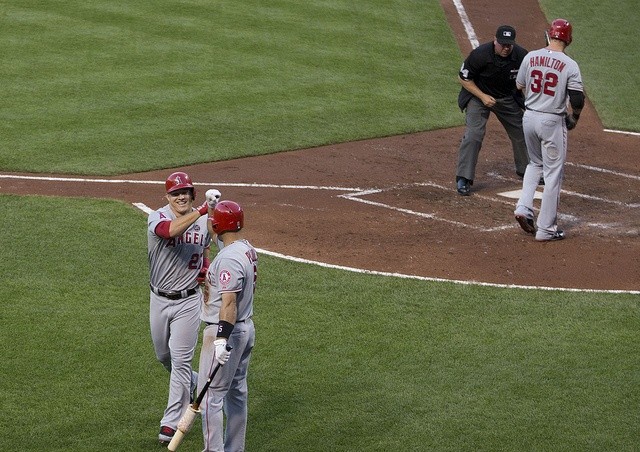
456,178,470,196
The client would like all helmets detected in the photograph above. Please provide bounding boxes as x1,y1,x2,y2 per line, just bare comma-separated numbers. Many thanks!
165,172,196,201
547,19,573,46
211,200,244,235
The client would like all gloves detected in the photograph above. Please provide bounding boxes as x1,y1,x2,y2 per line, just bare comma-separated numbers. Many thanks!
566,114,576,130
197,267,208,282
214,338,231,365
206,189,221,208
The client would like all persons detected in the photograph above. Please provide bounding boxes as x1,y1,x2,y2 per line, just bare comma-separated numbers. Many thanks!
514,17,585,244
202,188,259,451
146,170,219,443
456,23,532,197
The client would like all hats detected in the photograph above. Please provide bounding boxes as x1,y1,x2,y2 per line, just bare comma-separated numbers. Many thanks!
496,25,516,45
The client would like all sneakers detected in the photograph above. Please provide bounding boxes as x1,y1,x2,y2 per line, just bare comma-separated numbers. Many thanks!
515,207,536,234
516,171,544,185
191,372,198,402
535,231,566,242
158,425,176,441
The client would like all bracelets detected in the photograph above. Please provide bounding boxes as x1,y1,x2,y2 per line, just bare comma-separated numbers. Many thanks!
573,111,580,122
216,321,234,338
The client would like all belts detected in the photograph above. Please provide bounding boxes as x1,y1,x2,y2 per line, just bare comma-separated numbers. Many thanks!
205,320,245,326
525,106,562,116
150,283,200,300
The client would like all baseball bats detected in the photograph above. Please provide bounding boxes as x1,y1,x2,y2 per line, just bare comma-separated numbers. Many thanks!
168,344,232,452
